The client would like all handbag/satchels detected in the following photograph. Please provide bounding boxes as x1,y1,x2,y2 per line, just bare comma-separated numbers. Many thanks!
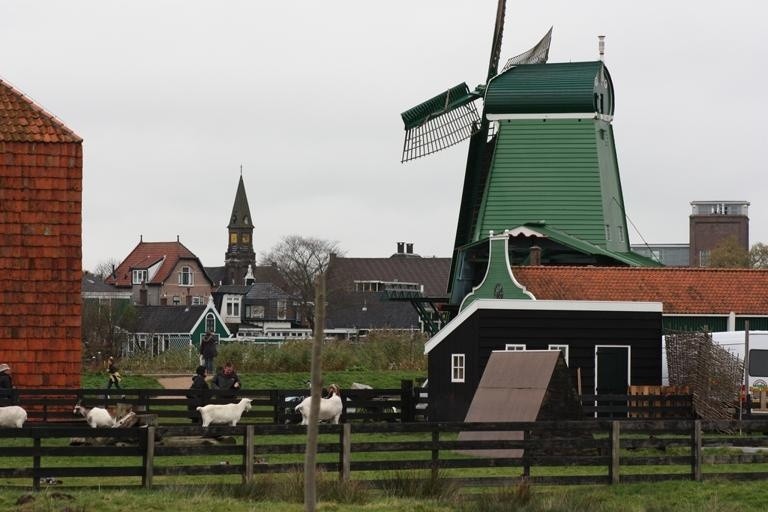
199,354,205,366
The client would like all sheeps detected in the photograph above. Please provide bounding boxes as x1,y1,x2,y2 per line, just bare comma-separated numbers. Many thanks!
283,379,344,426
0,404,29,428
72,399,114,428
196,397,254,428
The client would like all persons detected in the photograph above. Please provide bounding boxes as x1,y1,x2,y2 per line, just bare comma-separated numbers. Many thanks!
185,363,241,423
200,329,218,374
106,356,126,399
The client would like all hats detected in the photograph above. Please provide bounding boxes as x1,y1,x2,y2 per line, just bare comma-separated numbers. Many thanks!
0,363,10,372
